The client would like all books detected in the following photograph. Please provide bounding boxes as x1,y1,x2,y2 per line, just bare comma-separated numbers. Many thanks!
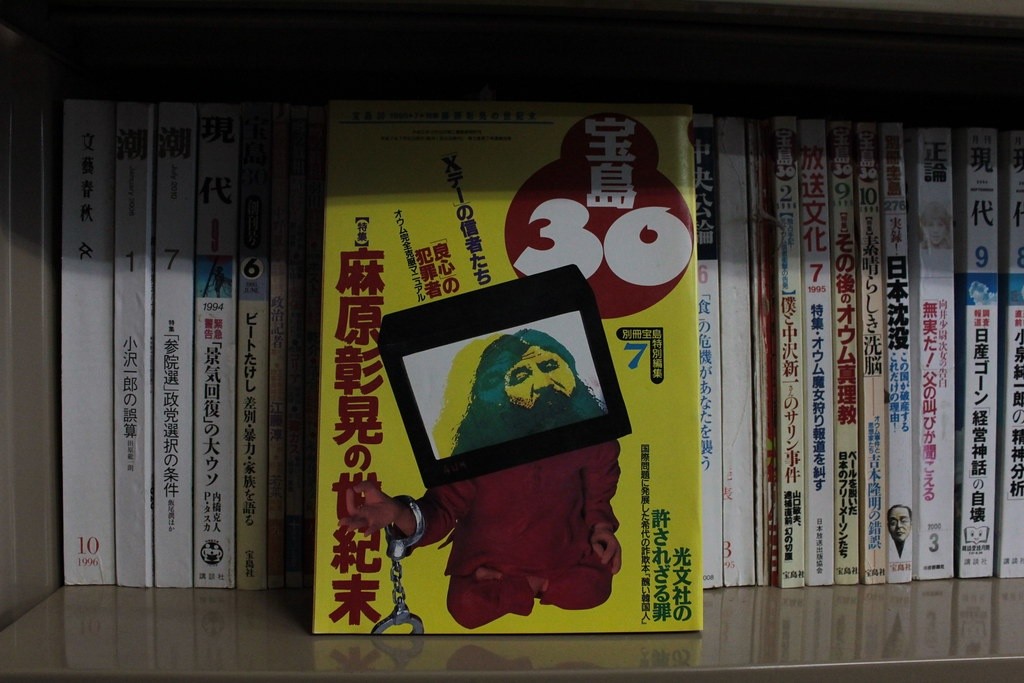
62,99,1024,634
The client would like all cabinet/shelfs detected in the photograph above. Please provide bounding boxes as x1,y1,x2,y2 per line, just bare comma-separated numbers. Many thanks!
0,0,1024,683
